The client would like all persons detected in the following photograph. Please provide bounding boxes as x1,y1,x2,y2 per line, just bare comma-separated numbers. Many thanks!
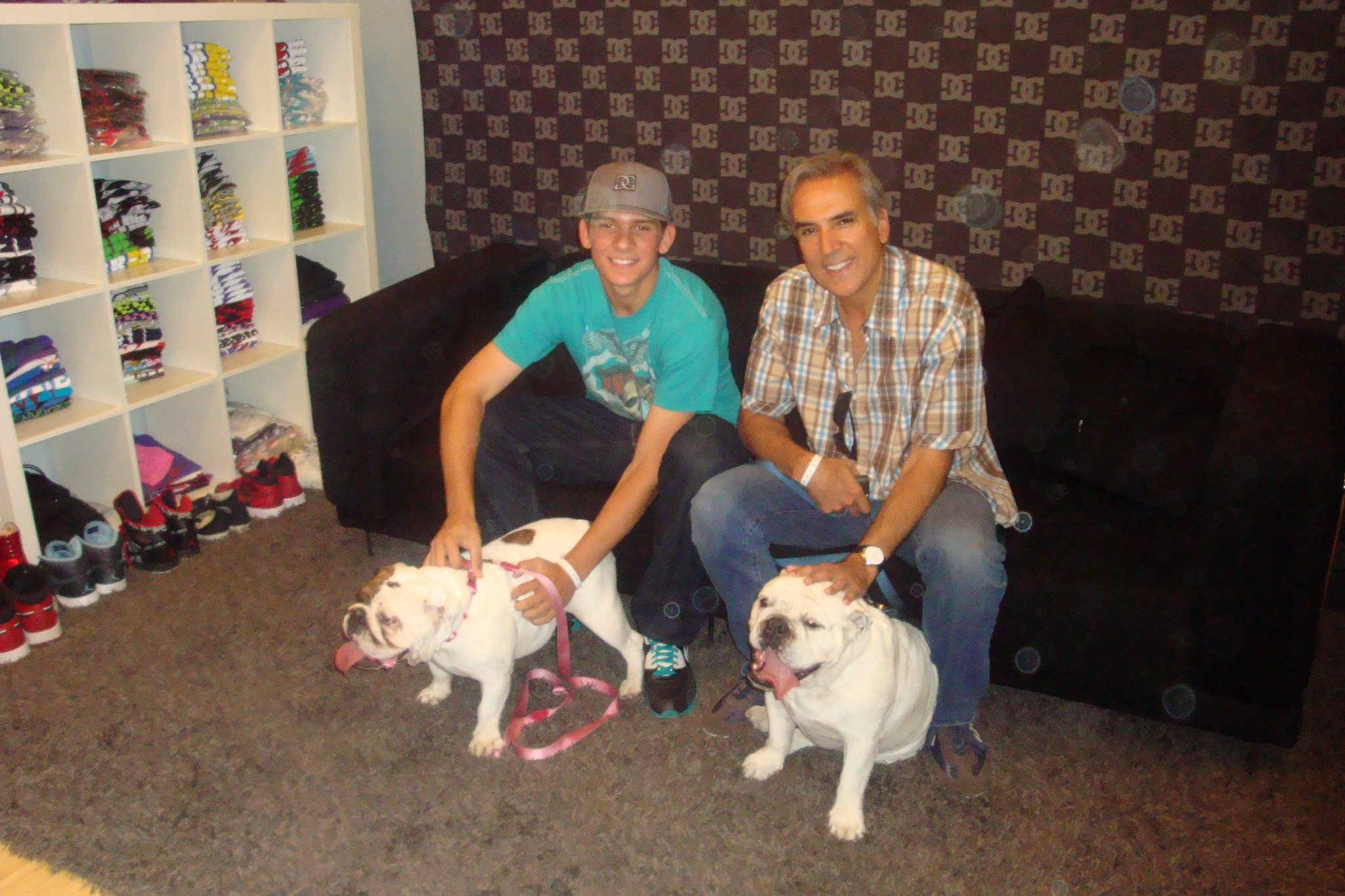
422,162,749,719
692,152,1018,791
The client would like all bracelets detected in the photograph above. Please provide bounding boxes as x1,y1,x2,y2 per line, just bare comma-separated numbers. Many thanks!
558,559,582,590
799,454,822,487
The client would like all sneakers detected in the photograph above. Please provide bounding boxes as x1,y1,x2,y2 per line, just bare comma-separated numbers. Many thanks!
0,563,63,645
701,674,768,738
153,488,201,558
209,488,252,532
0,601,29,664
35,534,99,610
930,720,989,799
74,519,128,595
269,452,307,507
215,465,289,518
113,489,180,575
191,498,231,541
641,631,697,719
550,610,583,641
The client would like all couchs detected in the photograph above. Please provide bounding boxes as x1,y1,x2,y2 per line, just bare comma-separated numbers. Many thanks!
302,241,1345,773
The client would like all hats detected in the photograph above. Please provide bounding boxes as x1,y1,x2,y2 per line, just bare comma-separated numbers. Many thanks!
579,162,671,221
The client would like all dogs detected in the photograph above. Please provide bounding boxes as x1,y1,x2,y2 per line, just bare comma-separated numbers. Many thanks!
738,567,942,845
330,515,646,760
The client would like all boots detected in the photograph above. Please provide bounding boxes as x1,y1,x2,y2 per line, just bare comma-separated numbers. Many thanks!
0,521,30,566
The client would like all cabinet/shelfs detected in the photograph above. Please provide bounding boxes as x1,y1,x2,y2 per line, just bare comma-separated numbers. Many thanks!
0,1,381,566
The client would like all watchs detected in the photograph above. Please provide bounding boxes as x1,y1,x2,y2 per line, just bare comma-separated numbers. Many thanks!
853,544,885,572
